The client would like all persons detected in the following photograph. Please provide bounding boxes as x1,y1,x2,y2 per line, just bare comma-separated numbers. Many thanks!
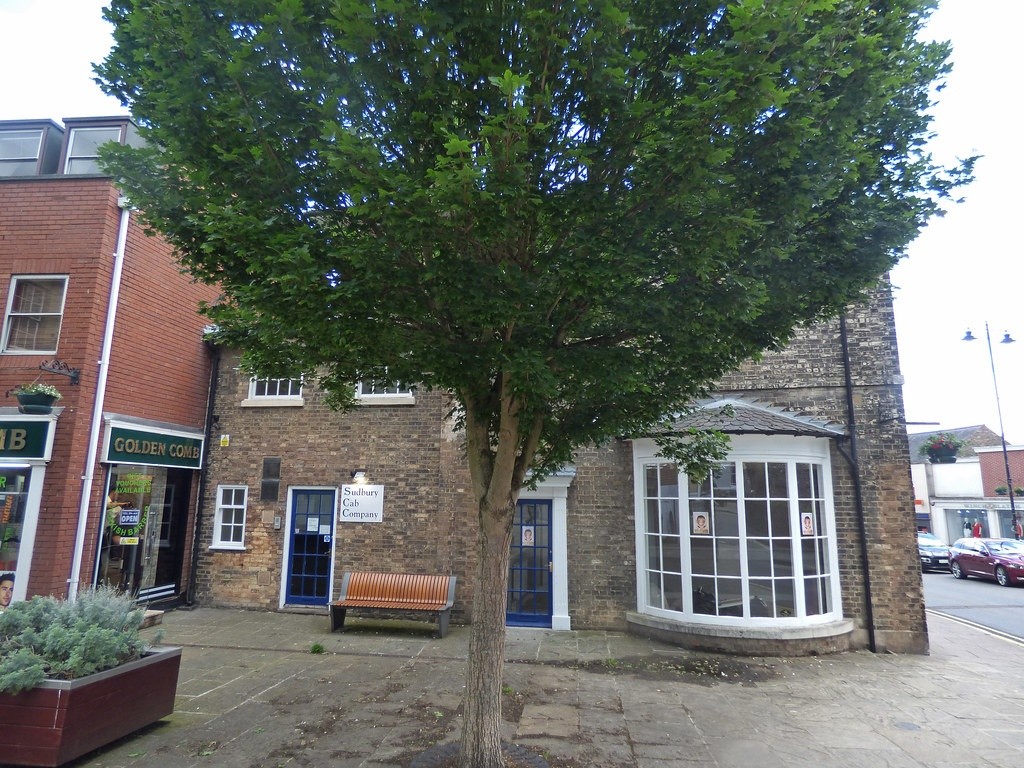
697,515,707,529
972,518,982,538
0,573,15,606
115,498,134,529
963,518,972,537
104,487,129,529
804,516,811,529
1011,520,1023,537
524,529,532,541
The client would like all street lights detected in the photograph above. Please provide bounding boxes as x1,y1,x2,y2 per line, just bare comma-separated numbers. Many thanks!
962,326,1021,539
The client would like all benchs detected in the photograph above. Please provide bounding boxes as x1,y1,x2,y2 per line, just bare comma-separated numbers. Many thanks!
327,572,456,639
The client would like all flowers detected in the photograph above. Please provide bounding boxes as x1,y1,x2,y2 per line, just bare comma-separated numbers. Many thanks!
918,432,967,456
5,383,64,400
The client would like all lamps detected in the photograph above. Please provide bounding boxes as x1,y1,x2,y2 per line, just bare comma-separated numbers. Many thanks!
350,467,368,480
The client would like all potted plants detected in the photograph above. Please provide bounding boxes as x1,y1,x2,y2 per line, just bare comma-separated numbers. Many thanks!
0,575,183,767
995,487,1008,495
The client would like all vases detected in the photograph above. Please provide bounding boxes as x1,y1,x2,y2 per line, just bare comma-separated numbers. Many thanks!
926,448,958,463
17,394,55,415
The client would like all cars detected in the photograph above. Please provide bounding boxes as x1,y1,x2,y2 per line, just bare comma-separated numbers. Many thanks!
948,536,1024,586
916,530,952,575
